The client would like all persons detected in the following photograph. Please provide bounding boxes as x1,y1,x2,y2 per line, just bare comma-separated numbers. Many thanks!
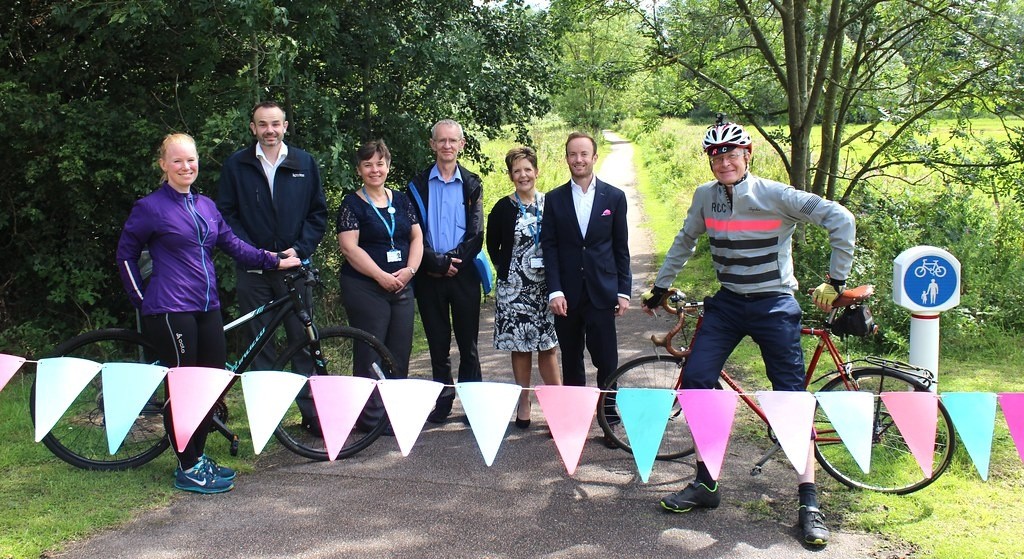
407,119,484,423
115,133,301,495
337,139,424,437
216,102,329,438
487,147,562,429
641,122,856,545
541,133,632,449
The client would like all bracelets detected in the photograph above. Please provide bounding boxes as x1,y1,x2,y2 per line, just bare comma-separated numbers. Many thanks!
406,266,416,275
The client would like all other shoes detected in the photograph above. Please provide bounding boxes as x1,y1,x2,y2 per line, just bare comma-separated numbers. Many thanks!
515,399,532,429
604,409,620,425
463,415,470,427
301,416,324,439
355,423,395,437
426,399,453,424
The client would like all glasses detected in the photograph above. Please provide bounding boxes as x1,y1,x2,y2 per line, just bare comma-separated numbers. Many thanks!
707,148,748,165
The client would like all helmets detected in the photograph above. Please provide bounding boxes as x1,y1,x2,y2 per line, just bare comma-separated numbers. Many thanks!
703,122,753,155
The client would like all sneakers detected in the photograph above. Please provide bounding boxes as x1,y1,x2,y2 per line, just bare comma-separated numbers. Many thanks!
174,460,234,494
174,452,236,481
661,481,720,513
796,505,830,545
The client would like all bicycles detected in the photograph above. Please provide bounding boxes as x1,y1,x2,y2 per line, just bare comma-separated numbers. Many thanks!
596,286,955,496
29,250,401,471
